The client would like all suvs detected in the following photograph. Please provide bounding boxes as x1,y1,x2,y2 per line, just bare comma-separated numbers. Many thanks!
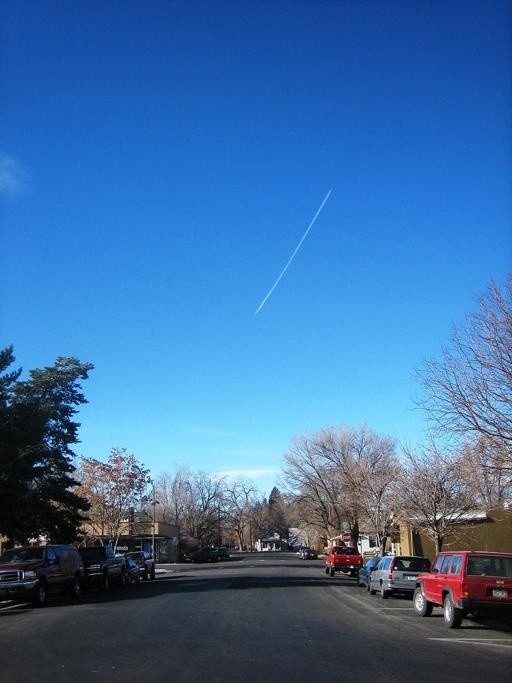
412,550,512,629
0,544,85,608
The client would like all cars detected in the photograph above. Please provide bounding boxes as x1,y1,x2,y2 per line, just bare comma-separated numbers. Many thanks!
299,547,317,561
369,556,431,599
183,547,231,564
78,545,156,593
357,555,382,592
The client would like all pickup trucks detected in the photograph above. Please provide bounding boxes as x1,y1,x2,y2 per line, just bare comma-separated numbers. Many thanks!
325,546,364,577
